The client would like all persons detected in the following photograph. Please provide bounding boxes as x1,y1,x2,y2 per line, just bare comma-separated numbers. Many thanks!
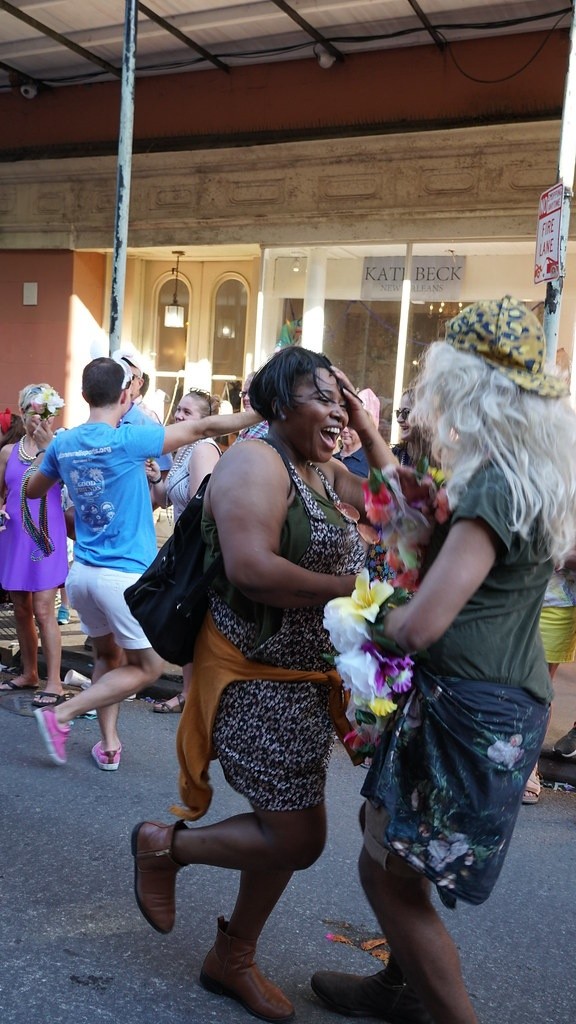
0,383,68,707
309,294,576,1024
331,408,379,478
389,386,446,488
130,346,401,1024
19,350,268,771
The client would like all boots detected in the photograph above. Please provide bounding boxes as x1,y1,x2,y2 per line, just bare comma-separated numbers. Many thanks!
200,916,295,1022
131,818,190,934
311,943,435,1024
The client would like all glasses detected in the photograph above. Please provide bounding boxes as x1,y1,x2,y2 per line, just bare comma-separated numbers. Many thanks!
334,502,380,548
131,373,142,381
238,390,247,398
189,387,211,416
396,409,411,420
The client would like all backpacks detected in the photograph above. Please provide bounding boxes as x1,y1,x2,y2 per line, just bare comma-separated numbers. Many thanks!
124,436,293,667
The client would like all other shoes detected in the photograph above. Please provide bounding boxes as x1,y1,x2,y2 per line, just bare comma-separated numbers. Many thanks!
84,636,93,650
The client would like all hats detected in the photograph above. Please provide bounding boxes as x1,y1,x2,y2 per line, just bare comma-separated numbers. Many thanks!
444,296,569,398
112,357,133,390
112,348,143,377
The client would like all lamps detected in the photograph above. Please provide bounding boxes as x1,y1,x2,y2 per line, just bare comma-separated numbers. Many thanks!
217,285,236,338
292,257,301,272
163,249,186,328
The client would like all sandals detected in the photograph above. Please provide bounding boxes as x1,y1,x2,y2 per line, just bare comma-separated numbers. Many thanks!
155,694,185,713
522,772,540,803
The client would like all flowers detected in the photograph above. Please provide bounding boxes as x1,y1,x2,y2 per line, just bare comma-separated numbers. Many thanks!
25,387,70,424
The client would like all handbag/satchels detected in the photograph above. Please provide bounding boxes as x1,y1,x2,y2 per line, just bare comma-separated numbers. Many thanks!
217,382,233,416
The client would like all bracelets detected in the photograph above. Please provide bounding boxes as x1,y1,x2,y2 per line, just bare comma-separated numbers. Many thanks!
19,467,55,561
35,448,48,458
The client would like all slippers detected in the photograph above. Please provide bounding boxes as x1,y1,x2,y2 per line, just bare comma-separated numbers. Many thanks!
0,678,39,691
31,691,65,707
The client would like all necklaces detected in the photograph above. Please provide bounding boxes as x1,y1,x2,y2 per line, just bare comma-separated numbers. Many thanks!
18,434,36,462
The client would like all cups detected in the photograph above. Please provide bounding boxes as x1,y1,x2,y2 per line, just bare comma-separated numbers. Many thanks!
64,669,91,686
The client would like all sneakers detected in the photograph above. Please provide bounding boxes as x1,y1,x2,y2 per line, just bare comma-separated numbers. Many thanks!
92,741,121,770
553,722,576,757
34,704,71,766
57,607,71,624
